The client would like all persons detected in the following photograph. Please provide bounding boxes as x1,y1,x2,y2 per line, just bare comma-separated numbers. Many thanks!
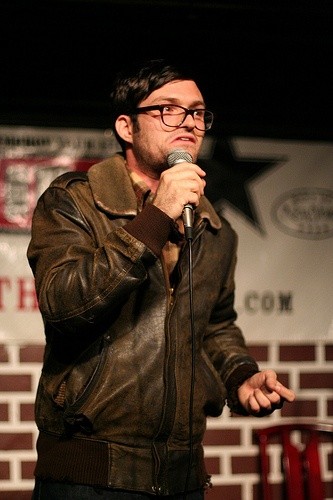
27,60,295,500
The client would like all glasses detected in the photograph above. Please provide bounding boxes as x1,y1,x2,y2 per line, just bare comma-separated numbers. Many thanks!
131,104,213,131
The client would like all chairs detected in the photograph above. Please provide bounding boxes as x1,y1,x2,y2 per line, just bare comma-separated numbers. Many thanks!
252,423,333,500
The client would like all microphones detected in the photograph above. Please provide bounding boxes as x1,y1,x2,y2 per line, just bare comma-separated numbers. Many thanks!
165,148,195,242
